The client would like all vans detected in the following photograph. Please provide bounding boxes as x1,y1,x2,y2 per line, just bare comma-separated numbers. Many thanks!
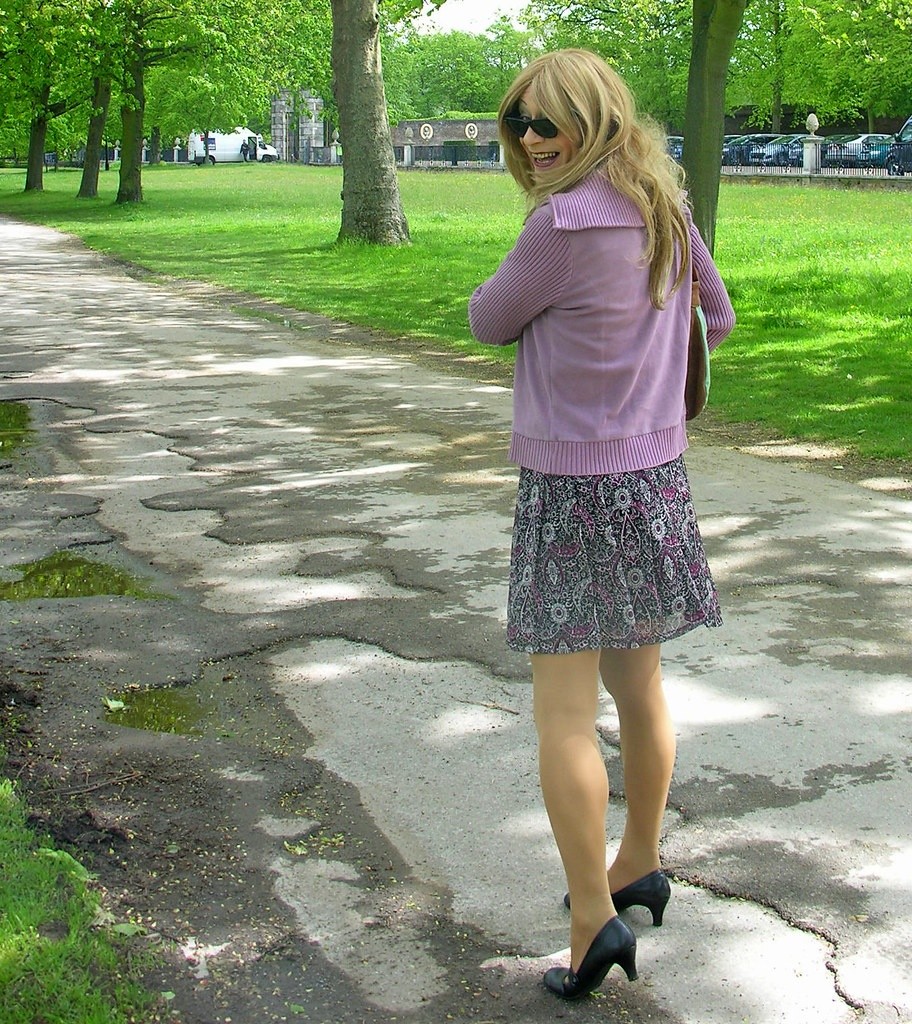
883,113,912,176
187,126,279,163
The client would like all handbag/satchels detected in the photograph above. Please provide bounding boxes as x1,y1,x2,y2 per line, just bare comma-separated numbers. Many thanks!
684,258,711,421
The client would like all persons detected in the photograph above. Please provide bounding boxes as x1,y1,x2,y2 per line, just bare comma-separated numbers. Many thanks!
466,48,735,1000
240,139,250,163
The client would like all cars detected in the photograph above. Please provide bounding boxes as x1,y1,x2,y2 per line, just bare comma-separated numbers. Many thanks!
666,136,684,162
824,133,894,168
722,134,743,150
747,133,834,167
794,133,851,168
721,133,787,165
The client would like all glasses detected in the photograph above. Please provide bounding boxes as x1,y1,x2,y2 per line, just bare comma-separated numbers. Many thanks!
502,109,558,138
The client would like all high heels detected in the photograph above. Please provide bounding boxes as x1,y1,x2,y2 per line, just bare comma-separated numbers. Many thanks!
563,865,672,926
542,914,639,1001
